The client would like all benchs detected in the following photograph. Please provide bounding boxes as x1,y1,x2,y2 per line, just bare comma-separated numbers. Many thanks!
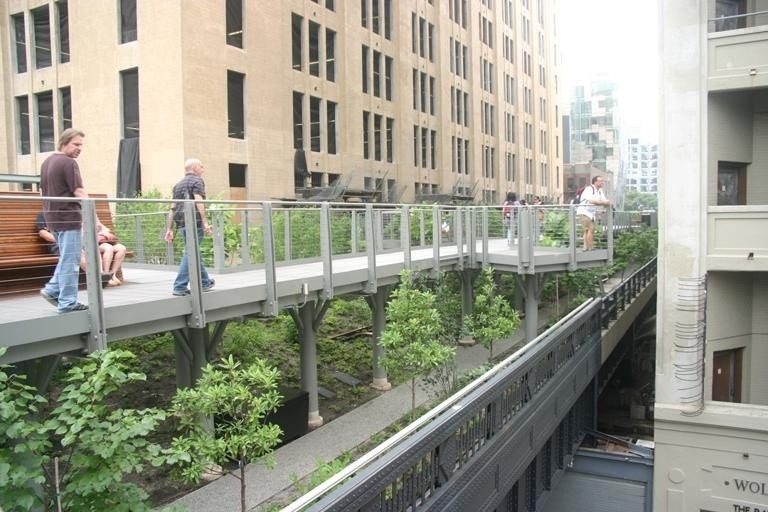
1,191,135,298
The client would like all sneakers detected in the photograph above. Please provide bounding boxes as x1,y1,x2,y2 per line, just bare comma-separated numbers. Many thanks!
41,290,88,313
203,278,215,292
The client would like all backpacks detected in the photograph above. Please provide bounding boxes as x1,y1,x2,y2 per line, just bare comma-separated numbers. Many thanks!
504,202,516,218
573,184,594,204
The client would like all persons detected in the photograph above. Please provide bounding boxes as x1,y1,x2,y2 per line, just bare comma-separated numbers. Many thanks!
164,157,216,297
575,174,611,252
517,199,529,206
531,196,548,242
80,210,127,287
35,127,103,315
502,191,521,248
36,210,115,290
